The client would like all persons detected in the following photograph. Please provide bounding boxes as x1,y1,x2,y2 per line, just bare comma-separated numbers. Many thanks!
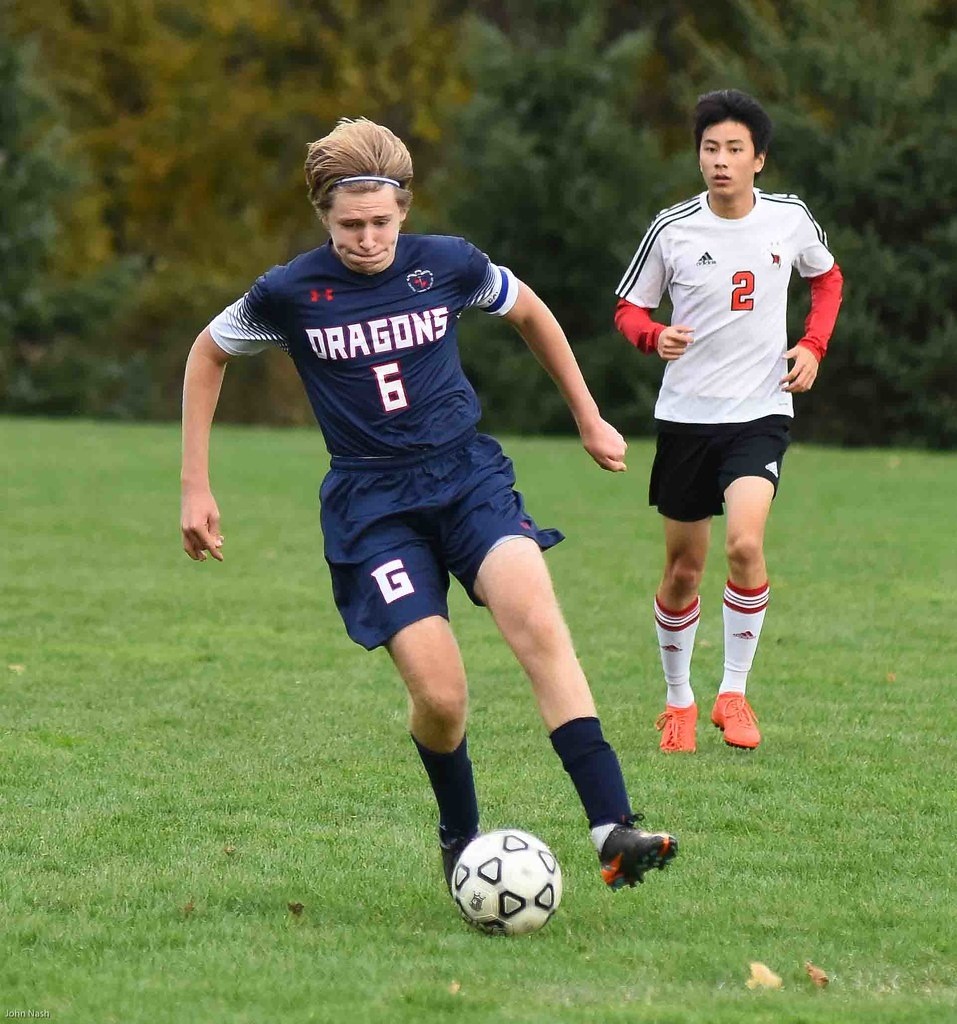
613,90,846,754
183,116,678,892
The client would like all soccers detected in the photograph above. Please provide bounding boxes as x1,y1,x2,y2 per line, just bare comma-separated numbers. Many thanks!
450,830,562,936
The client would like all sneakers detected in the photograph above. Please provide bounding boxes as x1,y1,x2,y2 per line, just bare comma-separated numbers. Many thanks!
599,813,677,893
654,702,698,754
711,691,760,749
438,823,480,899
